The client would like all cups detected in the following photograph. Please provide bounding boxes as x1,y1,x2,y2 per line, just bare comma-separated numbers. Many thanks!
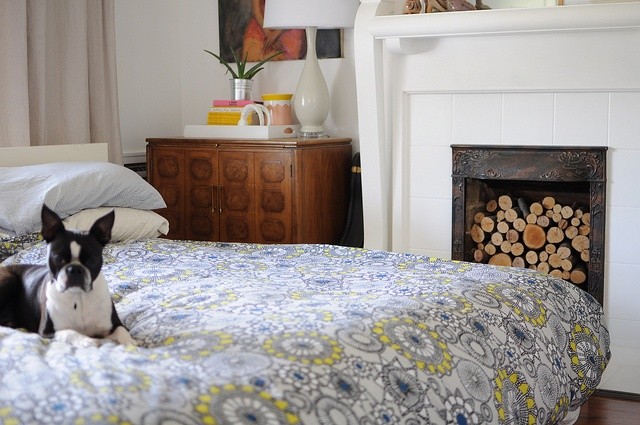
262,93,293,134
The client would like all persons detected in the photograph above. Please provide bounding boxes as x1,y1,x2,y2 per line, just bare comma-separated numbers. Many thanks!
242,0,303,62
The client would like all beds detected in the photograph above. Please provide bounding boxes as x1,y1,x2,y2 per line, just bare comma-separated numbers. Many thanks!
0,142,613,424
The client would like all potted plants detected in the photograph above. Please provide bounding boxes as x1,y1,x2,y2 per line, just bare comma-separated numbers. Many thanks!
204,45,285,100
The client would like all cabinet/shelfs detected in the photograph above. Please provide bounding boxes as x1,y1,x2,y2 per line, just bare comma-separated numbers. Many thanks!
145,137,352,245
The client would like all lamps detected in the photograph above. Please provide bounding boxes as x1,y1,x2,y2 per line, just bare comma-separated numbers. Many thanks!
263,0,360,138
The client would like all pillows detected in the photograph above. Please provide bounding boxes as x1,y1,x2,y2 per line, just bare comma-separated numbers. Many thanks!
0,162,167,234
62,206,170,239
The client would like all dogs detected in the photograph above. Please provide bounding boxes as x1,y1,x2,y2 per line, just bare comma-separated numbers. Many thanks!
0,203,140,347
